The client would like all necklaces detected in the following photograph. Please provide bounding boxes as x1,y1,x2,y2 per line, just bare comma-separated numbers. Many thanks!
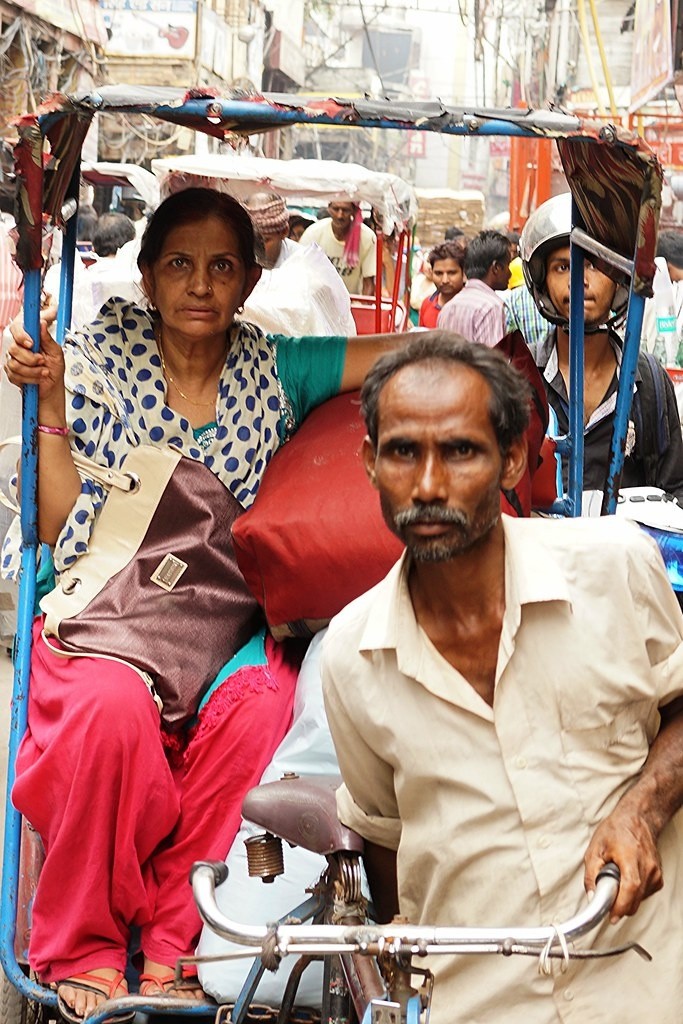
158,325,230,407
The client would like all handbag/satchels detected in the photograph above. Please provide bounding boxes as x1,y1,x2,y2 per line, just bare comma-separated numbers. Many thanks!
641,258,676,370
0,435,260,723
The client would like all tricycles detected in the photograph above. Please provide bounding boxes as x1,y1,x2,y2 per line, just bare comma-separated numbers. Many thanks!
0,84,682,1024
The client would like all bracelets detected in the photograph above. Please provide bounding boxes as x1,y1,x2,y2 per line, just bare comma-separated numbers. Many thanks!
37,425,69,436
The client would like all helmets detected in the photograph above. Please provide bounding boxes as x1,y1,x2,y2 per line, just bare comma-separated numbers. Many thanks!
518,190,633,336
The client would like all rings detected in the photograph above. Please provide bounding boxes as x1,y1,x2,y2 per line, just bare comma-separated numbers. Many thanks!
6,355,12,365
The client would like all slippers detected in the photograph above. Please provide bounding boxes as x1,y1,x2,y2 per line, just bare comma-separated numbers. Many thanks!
58,971,135,1024
140,974,203,996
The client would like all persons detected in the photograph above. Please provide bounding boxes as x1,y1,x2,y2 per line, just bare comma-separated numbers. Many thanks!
320,331,683,1024
3,188,444,1024
0,191,683,508
